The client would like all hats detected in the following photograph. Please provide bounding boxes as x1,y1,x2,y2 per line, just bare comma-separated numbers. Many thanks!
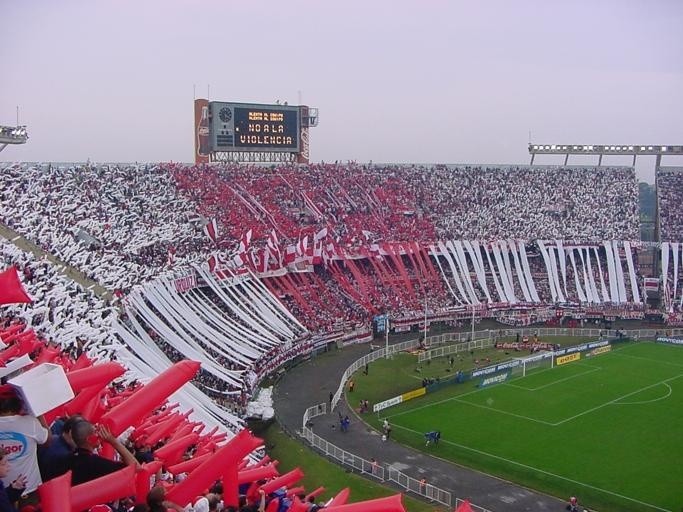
1,383,16,400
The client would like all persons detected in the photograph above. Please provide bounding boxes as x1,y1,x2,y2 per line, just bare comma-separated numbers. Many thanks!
1,161,683,512
